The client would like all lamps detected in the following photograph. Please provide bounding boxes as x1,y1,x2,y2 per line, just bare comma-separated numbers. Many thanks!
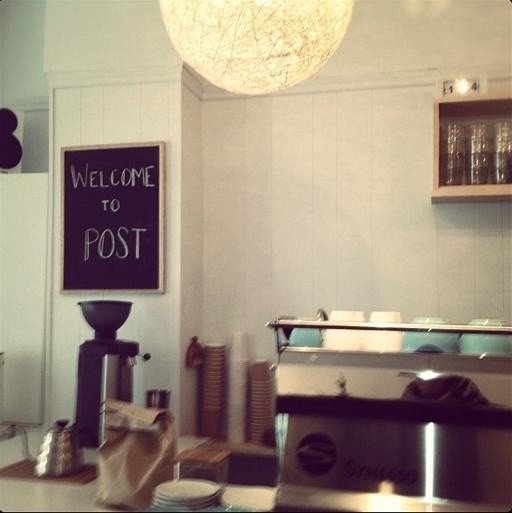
159,1,353,98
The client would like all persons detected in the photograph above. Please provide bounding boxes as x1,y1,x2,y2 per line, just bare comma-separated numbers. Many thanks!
399,344,489,405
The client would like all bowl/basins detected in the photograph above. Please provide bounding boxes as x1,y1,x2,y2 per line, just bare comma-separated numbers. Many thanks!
289,312,512,355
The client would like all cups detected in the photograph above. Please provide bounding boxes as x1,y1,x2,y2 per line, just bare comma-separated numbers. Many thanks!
201,333,275,447
146,388,170,409
441,119,510,183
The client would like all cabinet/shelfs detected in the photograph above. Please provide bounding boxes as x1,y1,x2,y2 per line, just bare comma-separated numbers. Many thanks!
432,89,512,202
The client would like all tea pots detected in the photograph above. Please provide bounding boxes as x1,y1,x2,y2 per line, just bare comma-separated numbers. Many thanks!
11,419,84,477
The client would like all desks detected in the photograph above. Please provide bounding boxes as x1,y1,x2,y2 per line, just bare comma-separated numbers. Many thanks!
267,318,512,512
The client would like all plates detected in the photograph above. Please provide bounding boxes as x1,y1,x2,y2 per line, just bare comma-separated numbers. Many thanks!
150,479,227,510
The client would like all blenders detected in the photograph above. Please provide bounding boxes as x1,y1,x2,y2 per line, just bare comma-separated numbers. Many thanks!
77,300,139,448
